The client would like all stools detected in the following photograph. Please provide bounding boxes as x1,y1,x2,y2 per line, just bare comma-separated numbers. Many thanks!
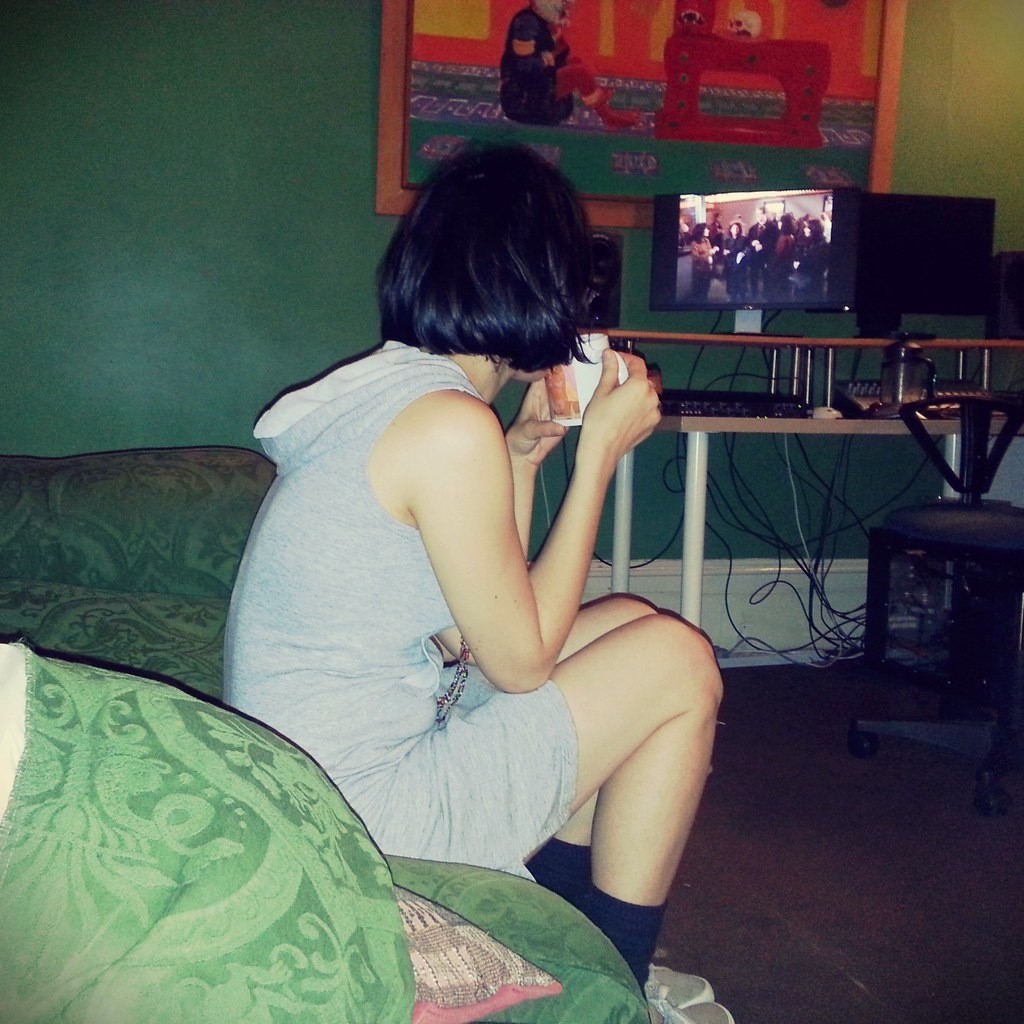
850,502,1024,817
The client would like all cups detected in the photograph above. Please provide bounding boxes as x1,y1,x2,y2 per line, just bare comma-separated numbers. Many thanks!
544,333,629,427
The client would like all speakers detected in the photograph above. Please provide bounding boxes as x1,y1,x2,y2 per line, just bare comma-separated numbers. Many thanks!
578,230,623,331
983,251,1024,341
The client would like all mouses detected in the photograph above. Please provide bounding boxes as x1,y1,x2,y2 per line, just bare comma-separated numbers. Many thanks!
813,406,842,419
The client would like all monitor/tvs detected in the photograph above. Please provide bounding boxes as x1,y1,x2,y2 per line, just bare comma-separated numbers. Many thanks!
807,193,995,343
649,185,856,338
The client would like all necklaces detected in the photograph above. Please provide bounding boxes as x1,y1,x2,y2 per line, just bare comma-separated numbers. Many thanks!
435,637,473,722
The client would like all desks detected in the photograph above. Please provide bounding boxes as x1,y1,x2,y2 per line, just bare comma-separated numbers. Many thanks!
575,323,1024,629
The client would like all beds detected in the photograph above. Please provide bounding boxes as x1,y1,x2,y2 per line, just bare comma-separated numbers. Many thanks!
0,446,647,1024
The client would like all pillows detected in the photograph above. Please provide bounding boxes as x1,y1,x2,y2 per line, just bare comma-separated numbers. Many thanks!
394,884,564,1024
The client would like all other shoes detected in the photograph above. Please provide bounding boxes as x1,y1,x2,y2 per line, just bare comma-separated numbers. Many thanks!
644,963,716,1009
646,999,734,1024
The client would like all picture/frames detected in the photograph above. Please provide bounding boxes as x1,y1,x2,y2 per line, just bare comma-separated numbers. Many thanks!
372,0,906,230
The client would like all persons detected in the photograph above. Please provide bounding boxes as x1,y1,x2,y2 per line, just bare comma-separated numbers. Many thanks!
219,147,735,1024
691,207,830,302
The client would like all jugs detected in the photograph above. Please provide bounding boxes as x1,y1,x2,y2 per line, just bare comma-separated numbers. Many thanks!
875,332,940,419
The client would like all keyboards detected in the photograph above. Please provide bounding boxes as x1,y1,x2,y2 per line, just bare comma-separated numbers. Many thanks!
657,388,813,420
835,377,995,420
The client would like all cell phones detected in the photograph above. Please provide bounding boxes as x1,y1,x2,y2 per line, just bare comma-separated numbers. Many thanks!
893,330,939,343
